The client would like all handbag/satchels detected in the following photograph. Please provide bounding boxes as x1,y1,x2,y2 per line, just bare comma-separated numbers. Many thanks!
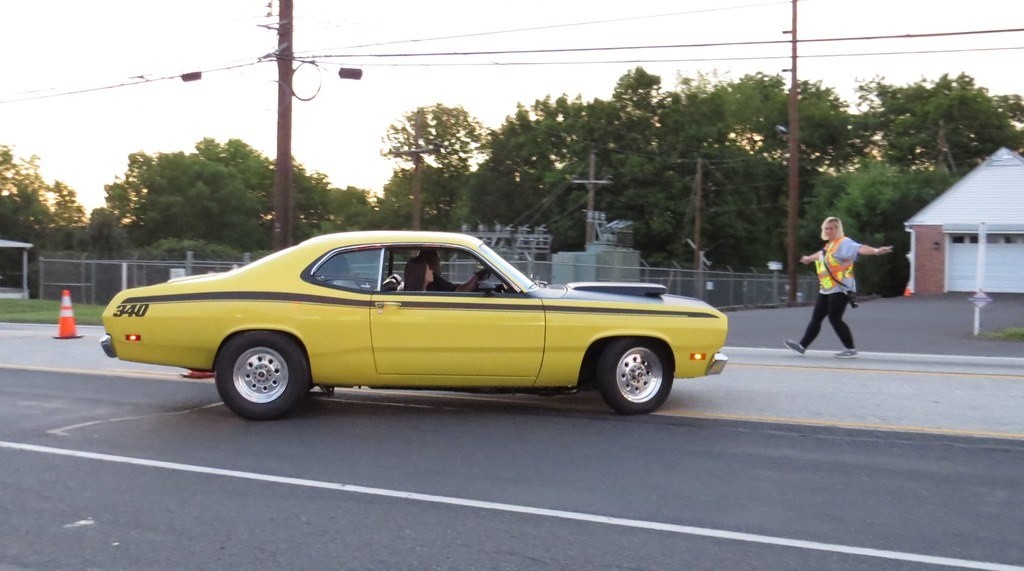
847,290,859,309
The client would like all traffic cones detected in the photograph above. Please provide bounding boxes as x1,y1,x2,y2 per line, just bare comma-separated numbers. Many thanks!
51,288,82,341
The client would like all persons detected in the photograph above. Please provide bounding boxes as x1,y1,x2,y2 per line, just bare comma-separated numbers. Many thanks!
784,216,893,359
419,248,491,293
403,255,434,292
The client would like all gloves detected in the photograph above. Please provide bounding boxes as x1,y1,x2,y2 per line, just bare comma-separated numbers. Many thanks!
473,269,491,281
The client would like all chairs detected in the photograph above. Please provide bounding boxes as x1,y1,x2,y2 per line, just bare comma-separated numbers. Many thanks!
379,272,405,292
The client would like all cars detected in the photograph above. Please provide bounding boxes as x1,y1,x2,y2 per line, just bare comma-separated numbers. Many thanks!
98,228,732,415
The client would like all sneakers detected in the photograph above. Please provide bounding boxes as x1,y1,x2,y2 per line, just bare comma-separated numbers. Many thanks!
784,339,805,356
835,348,857,359
